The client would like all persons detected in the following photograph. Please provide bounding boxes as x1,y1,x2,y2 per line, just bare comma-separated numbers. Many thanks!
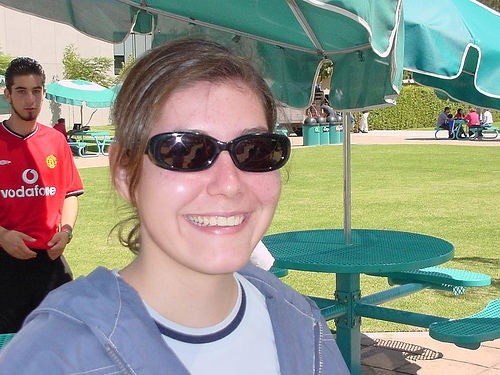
0,33,351,375
0,57,84,334
53,118,67,141
359,110,370,133
437,107,493,140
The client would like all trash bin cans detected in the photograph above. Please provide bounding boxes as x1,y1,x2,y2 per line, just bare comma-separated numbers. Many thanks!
302,115,345,146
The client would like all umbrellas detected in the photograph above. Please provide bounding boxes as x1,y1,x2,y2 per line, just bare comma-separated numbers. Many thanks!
0,85,13,119
45,79,116,142
0,0,404,245
403,0,500,112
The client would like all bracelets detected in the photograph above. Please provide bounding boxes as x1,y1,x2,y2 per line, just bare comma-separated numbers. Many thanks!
62,224,73,244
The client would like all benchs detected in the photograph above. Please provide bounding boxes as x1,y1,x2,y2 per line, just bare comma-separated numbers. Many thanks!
470,127,499,140
435,128,444,140
80,140,115,156
68,142,86,158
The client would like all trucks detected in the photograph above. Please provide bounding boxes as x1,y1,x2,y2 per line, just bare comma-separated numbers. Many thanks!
276,90,356,137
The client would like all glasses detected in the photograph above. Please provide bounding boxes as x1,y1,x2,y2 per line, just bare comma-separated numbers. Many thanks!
143,131,292,173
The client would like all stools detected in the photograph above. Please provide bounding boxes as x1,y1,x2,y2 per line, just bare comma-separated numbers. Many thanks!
364,267,492,287
429,299,500,345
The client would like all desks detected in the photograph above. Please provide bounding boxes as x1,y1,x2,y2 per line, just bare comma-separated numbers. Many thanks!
261,229,454,374
455,120,469,140
67,132,109,158
